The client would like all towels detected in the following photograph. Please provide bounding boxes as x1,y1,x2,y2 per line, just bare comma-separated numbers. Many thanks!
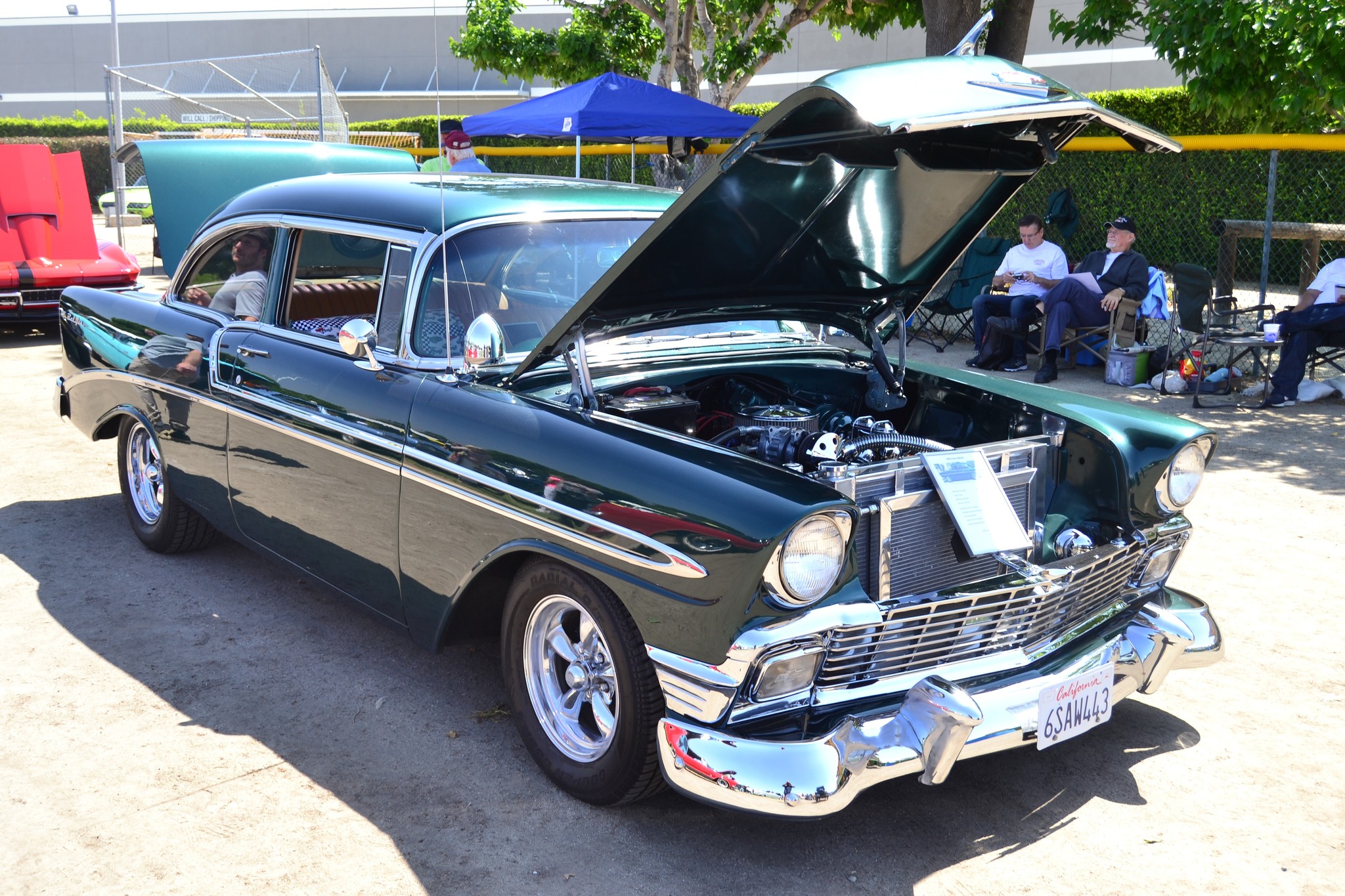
1140,266,1170,321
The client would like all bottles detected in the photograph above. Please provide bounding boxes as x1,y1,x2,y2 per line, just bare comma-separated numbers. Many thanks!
1178,354,1185,379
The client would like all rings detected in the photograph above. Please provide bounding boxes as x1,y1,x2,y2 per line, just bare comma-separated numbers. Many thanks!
1028,277,1030,279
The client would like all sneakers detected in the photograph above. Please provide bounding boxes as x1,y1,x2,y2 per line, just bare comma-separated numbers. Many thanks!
1257,317,1279,330
965,354,982,367
1003,358,1028,372
1271,394,1295,407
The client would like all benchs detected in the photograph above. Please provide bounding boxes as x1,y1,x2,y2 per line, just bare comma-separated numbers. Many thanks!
288,303,572,358
284,274,518,329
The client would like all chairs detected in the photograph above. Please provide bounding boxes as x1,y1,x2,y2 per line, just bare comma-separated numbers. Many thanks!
1036,261,1170,376
968,255,1084,366
900,235,1014,354
1161,261,1277,409
1284,281,1345,381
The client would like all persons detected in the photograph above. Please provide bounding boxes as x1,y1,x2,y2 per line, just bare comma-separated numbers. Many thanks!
185,233,271,323
418,119,492,175
966,215,1068,372
119,335,207,443
988,217,1148,383
1260,258,1345,407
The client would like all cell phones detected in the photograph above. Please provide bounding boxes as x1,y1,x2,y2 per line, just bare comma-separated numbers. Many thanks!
1334,284,1345,303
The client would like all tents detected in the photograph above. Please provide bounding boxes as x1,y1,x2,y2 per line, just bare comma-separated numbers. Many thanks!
461,73,760,184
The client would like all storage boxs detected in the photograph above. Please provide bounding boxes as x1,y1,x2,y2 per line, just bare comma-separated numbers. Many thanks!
1185,375,1243,392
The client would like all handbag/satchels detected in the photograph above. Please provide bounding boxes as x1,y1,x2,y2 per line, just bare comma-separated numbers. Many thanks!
1147,345,1173,380
1106,351,1148,384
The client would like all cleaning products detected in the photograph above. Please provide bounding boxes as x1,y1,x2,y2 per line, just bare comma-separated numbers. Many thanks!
1187,351,1205,381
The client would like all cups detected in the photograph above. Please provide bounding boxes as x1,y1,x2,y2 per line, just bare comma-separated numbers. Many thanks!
1263,324,1280,342
1232,366,1242,378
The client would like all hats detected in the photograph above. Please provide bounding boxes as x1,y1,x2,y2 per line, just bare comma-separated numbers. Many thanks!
1103,216,1136,233
440,130,472,150
441,119,463,134
248,229,269,243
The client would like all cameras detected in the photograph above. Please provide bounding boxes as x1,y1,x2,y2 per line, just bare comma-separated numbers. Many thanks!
1011,273,1025,280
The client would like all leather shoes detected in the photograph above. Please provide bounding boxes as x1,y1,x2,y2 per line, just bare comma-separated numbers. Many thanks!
1033,363,1058,383
987,315,1029,341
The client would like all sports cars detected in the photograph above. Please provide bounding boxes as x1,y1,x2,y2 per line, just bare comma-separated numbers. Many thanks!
52,8,1224,818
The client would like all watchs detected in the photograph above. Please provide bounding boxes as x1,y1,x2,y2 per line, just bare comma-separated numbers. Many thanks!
1119,287,1125,295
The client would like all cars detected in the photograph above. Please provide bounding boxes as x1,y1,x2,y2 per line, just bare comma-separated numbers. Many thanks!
0,144,146,343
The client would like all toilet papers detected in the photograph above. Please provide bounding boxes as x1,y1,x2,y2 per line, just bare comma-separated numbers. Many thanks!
1204,367,1228,383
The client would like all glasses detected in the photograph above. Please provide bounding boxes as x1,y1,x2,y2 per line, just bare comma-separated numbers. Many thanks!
1107,229,1118,236
1020,230,1039,238
443,151,447,155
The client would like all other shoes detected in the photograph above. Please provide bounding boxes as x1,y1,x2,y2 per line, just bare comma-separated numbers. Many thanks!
160,429,191,444
